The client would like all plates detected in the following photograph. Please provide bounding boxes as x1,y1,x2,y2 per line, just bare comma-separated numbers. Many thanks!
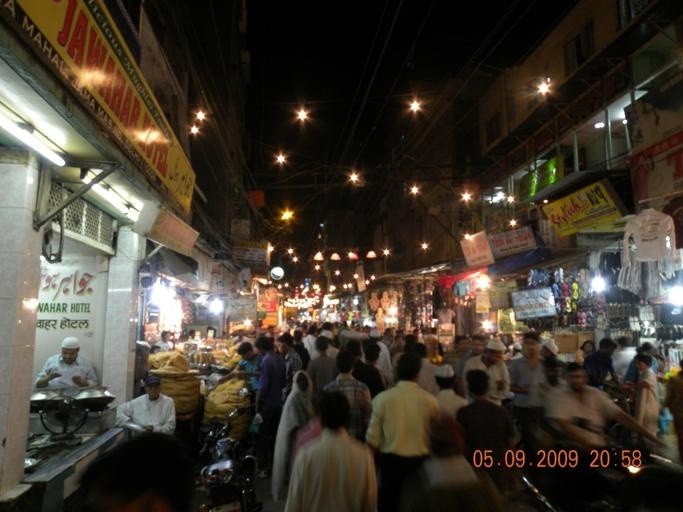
489,390,514,399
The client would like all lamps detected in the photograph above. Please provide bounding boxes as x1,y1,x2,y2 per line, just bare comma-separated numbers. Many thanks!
79,166,139,222
0,110,67,168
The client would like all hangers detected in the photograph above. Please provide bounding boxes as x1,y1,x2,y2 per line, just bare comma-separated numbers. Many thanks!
644,201,650,209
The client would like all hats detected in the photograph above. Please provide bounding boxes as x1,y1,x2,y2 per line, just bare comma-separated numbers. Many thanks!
367,328,381,338
485,338,507,352
144,375,162,385
433,364,455,380
61,336,80,350
542,341,559,358
318,329,334,340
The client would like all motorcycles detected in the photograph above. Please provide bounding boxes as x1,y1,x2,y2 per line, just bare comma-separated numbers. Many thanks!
193,388,263,512
521,421,681,512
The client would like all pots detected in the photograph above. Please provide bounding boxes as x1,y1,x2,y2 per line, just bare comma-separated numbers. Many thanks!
70,396,114,412
30,400,64,415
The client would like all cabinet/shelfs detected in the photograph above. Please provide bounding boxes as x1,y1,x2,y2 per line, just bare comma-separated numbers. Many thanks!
554,331,593,353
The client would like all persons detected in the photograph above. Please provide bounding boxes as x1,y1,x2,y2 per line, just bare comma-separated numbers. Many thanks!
114,320,682,511
35,336,98,387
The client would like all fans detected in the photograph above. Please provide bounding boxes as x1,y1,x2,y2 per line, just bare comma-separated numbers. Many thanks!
40,396,88,434
